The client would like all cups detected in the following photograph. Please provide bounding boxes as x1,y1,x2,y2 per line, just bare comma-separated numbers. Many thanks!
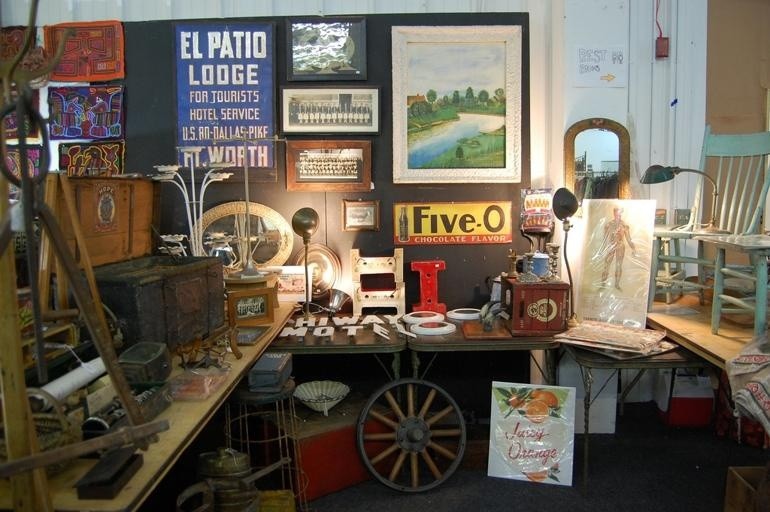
485,272,510,305
531,253,549,277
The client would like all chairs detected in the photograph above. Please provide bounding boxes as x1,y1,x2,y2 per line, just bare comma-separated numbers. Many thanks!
647,124,769,314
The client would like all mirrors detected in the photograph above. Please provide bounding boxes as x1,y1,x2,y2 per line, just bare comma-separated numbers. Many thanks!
563,118,629,199
189,202,294,278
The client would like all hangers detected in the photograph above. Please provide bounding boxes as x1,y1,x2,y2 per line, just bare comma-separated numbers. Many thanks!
575,169,618,179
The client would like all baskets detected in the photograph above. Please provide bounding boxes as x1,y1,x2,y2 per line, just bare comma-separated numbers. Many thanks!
293,379,351,413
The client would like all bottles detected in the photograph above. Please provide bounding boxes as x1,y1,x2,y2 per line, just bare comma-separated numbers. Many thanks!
399,206,409,242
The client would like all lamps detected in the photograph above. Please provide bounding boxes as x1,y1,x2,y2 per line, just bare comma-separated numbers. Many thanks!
640,164,731,234
553,187,581,328
292,207,320,319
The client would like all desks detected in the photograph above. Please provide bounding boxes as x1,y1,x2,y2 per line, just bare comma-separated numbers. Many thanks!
647,287,770,369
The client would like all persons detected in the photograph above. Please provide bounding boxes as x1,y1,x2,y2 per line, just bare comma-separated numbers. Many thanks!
600,208,637,291
306,253,329,292
295,101,370,124
300,153,362,180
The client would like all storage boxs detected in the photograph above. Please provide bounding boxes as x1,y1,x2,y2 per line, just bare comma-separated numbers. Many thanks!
92,254,224,349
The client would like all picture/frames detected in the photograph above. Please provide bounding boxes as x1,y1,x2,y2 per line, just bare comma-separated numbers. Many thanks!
295,244,342,297
170,19,277,184
285,16,368,81
391,24,522,183
285,139,372,194
341,199,381,232
278,86,382,134
262,265,313,303
